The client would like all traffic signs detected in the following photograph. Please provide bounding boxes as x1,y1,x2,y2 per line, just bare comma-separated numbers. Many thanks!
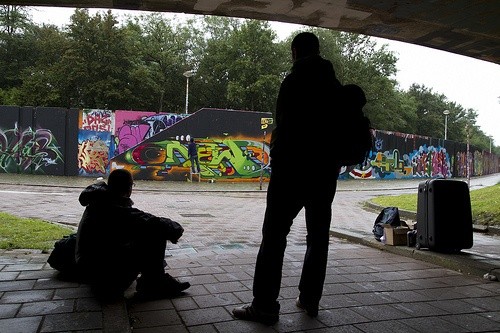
259,116,273,130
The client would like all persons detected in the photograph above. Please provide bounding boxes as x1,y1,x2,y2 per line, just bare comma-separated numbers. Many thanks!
232,32,348,322
75,169,191,298
188,138,201,173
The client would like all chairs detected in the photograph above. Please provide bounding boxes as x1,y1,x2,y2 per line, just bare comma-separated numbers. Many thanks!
190,167,200,182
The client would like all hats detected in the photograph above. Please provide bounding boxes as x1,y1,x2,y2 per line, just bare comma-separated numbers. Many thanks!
108,169,134,187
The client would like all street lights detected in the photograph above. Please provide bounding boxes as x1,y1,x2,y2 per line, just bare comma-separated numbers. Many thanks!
442,108,451,140
182,66,199,115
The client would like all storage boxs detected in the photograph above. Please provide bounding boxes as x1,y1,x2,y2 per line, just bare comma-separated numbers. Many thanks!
381,224,408,245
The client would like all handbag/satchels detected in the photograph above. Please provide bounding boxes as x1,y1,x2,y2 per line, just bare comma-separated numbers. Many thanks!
47,233,87,273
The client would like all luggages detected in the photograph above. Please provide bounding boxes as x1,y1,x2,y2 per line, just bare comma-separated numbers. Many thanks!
416,177,473,254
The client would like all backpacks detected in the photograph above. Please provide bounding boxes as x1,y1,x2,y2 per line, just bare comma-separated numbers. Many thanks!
373,207,400,242
318,78,372,174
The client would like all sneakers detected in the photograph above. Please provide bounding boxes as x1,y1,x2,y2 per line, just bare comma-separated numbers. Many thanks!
298,296,318,316
233,300,280,325
142,273,189,291
